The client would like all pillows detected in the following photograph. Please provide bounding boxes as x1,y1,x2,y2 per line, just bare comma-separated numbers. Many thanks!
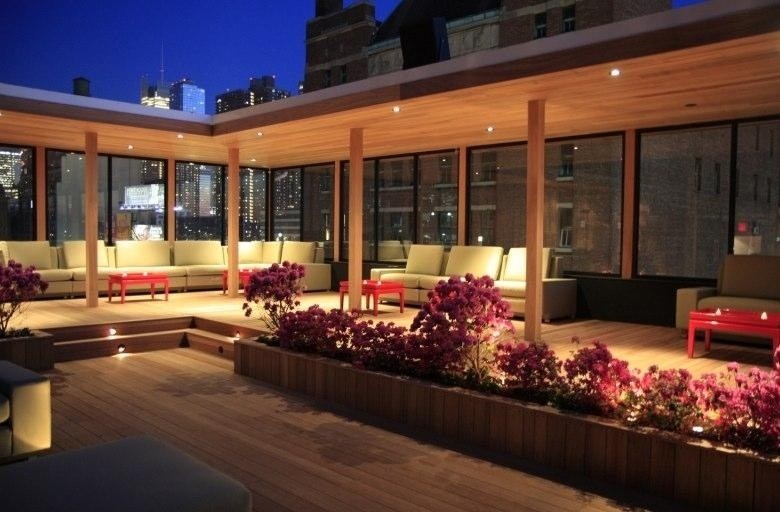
445,246,503,281
115,240,170,266
406,244,444,276
503,247,553,283
237,241,263,264
281,241,316,263
63,239,108,269
174,239,224,267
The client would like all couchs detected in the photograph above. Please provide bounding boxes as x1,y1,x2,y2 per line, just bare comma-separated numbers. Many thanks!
57,240,170,298
676,255,780,339
0,240,73,304
372,244,449,304
170,241,332,293
0,359,53,467
1,434,253,511
419,245,578,322
